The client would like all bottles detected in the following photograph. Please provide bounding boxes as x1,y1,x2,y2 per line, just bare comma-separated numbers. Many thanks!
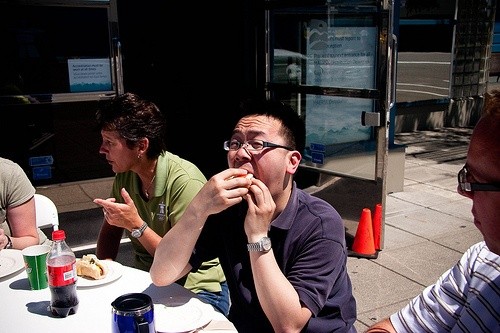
46,230,79,317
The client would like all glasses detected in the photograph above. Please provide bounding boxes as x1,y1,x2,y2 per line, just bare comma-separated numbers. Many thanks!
223,139,295,152
457,164,499,192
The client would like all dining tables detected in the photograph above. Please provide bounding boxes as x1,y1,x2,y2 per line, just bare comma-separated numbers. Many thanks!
0,258,240,333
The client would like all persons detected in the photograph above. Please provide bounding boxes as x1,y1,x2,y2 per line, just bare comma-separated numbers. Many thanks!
149,99,359,333
365,105,500,333
0,157,52,251
95,92,228,319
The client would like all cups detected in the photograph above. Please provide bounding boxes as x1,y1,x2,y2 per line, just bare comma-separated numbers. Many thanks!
111,292,155,333
22,245,51,290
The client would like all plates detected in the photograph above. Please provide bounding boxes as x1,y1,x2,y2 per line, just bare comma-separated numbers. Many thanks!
0,247,26,279
74,260,123,287
152,296,213,333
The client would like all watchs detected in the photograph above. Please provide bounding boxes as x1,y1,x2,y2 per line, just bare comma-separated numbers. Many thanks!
246,237,272,254
131,220,148,239
2,234,13,249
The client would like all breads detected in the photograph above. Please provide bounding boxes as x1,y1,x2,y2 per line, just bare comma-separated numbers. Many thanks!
227,171,254,185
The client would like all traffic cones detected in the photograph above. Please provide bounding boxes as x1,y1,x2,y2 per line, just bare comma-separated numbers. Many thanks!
347,208,378,259
372,203,382,251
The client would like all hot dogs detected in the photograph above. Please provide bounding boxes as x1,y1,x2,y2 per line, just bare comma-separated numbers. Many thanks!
76,254,106,279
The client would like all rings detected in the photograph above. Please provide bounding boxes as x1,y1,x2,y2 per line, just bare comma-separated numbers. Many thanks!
104,212,107,216
